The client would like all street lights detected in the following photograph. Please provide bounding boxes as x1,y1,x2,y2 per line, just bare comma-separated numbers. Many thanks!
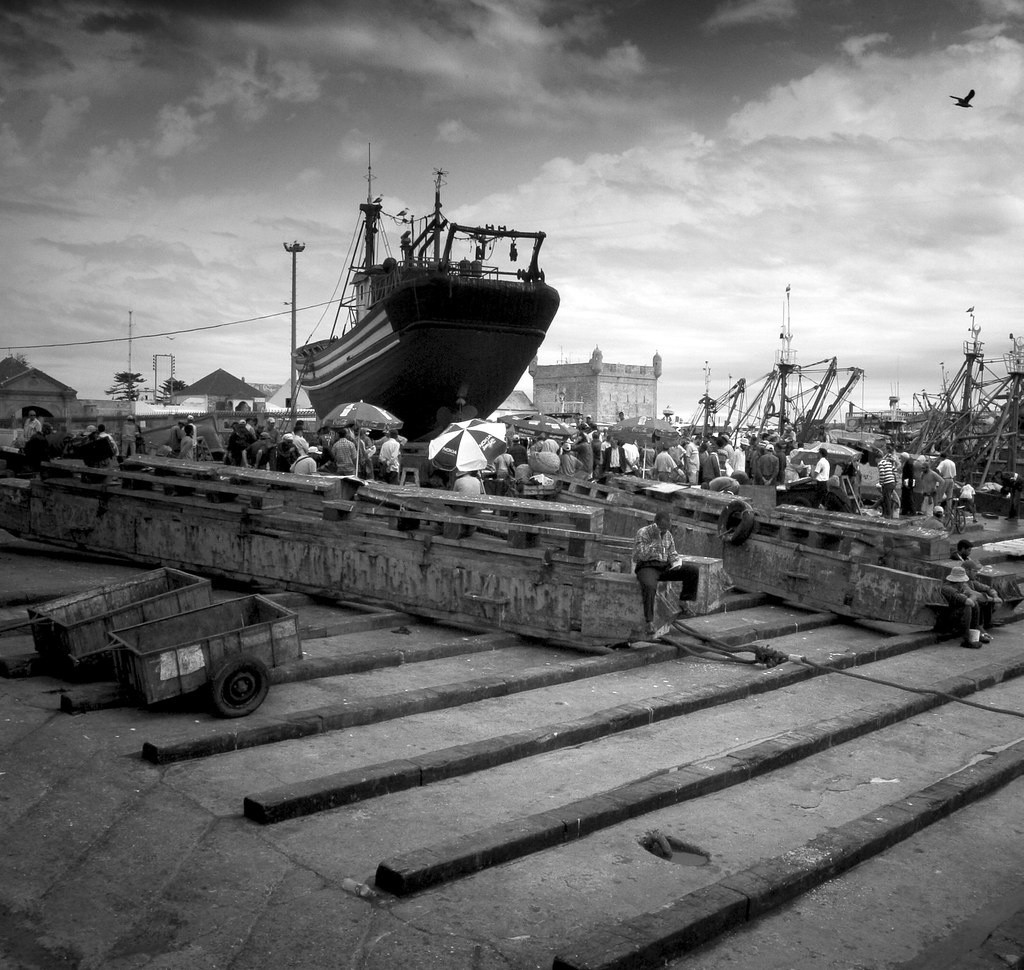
283,240,306,430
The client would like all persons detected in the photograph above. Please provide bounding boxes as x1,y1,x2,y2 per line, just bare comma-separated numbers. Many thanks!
993,471,1022,520
633,511,699,635
23,410,408,484
452,412,978,531
941,538,1003,649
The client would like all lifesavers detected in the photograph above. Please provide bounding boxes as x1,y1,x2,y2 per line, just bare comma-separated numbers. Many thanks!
559,392,566,403
764,402,776,420
845,418,860,431
717,500,756,546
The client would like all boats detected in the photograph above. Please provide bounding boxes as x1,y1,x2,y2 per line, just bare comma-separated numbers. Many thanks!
291,141,561,453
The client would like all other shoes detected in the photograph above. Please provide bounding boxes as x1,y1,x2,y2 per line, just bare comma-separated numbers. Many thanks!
973,519,977,522
645,622,655,634
978,633,990,643
678,602,696,618
983,623,993,630
960,639,982,648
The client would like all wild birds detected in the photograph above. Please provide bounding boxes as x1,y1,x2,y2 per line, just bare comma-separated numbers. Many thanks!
966,306,975,312
949,89,975,108
786,283,791,292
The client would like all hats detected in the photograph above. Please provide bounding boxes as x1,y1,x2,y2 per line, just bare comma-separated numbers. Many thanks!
933,505,944,514
267,417,276,423
586,415,591,419
901,452,910,459
282,434,293,441
562,443,572,451
260,432,272,439
83,425,96,436
765,444,775,451
239,419,246,425
945,566,970,582
961,560,981,570
308,446,323,454
740,438,751,447
188,414,193,420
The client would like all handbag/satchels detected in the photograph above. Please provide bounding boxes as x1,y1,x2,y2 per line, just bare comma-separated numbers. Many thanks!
507,468,513,475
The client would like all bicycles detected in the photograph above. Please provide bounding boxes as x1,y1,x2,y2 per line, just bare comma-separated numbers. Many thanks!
937,497,967,534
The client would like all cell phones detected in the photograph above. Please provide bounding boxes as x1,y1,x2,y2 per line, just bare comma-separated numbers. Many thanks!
653,523,657,527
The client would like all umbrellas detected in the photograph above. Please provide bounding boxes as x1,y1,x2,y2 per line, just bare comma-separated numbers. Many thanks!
496,412,580,441
607,416,681,479
790,441,863,466
428,418,507,471
321,399,404,476
837,431,891,453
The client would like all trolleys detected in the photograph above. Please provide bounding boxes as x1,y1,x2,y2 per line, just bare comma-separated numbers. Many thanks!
67,593,306,718
0,568,214,666
